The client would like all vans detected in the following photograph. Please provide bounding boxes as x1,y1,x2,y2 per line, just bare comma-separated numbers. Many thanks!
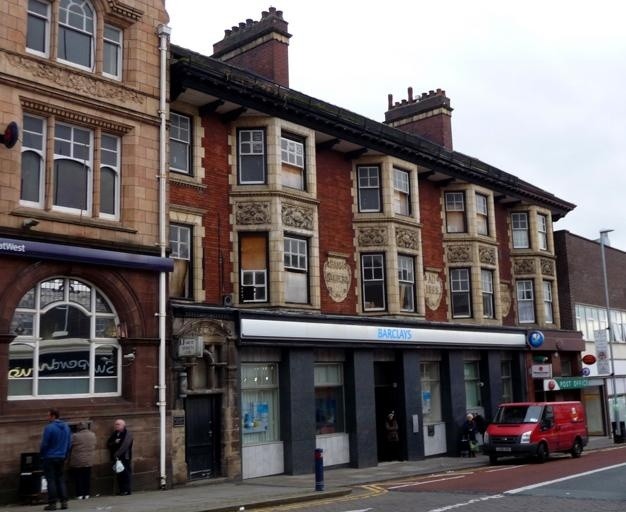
484,397,589,460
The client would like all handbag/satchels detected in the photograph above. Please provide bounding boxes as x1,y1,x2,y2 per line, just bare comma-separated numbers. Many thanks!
112,456,125,473
468,440,480,452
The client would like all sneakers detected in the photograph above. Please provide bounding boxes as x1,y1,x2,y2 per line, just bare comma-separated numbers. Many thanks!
44,504,57,510
61,501,67,509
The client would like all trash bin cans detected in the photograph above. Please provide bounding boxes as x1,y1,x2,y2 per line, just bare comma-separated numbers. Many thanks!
612,421,626,443
19,451,46,504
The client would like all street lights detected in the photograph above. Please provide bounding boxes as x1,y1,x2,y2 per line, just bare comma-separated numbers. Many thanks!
600,229,623,441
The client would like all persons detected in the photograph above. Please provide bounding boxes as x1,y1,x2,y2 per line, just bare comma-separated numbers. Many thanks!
72,421,96,500
385,410,399,443
106,418,133,495
463,412,476,458
40,408,72,510
